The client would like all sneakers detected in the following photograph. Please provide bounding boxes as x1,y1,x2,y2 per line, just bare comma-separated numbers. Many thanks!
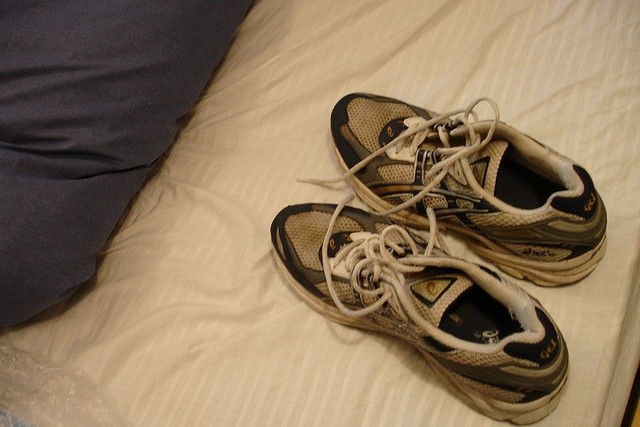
330,92,608,288
269,202,570,426
